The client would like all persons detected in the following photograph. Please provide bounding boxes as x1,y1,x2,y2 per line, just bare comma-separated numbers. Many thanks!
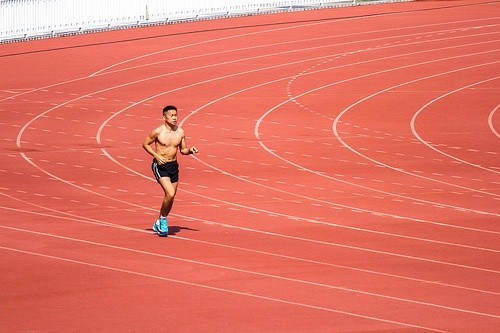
142,106,198,236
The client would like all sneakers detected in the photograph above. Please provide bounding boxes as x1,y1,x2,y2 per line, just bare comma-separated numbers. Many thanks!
152,223,168,236
156,215,168,235
